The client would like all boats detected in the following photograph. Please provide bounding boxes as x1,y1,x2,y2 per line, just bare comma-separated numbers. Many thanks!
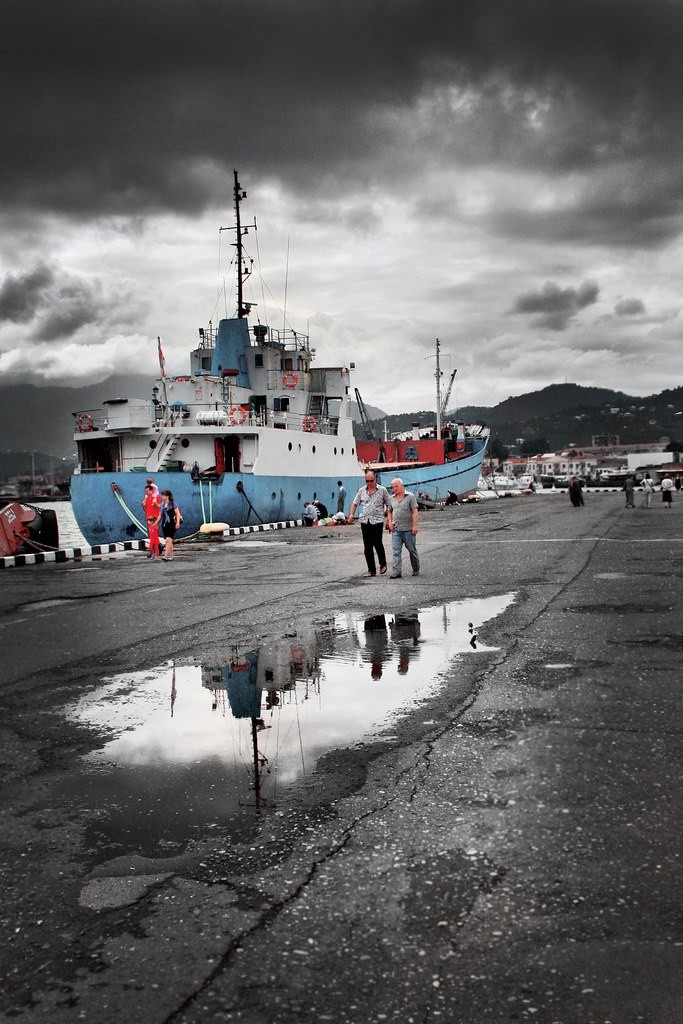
0,495,62,558
66,168,648,547
199,617,336,824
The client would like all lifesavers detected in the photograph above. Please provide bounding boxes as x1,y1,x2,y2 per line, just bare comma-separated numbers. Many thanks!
302,416,316,432
78,414,94,431
283,371,298,387
229,406,247,425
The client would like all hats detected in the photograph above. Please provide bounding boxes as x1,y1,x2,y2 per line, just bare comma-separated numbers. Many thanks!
313,500,318,505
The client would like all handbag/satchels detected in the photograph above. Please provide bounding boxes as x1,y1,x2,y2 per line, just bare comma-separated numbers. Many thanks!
652,488,655,493
661,487,663,491
172,505,183,524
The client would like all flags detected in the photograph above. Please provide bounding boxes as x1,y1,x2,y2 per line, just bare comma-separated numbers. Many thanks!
158,336,168,384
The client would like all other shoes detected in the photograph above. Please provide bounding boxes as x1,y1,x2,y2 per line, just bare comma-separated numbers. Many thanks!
412,565,419,576
390,573,401,578
147,552,151,557
363,571,376,577
152,503,155,506
154,557,158,561
168,557,174,561
380,566,386,574
161,556,169,562
159,543,164,554
151,555,154,560
143,503,145,507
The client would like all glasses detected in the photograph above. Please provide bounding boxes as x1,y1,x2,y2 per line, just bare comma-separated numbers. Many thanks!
366,478,375,482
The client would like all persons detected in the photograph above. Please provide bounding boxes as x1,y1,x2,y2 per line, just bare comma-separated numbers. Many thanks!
529,482,536,494
674,474,681,497
142,477,180,563
386,478,420,579
623,474,635,508
362,608,420,681
347,470,391,577
302,499,328,527
417,491,435,511
661,474,674,508
445,490,457,506
569,474,584,507
337,480,346,513
639,474,653,509
